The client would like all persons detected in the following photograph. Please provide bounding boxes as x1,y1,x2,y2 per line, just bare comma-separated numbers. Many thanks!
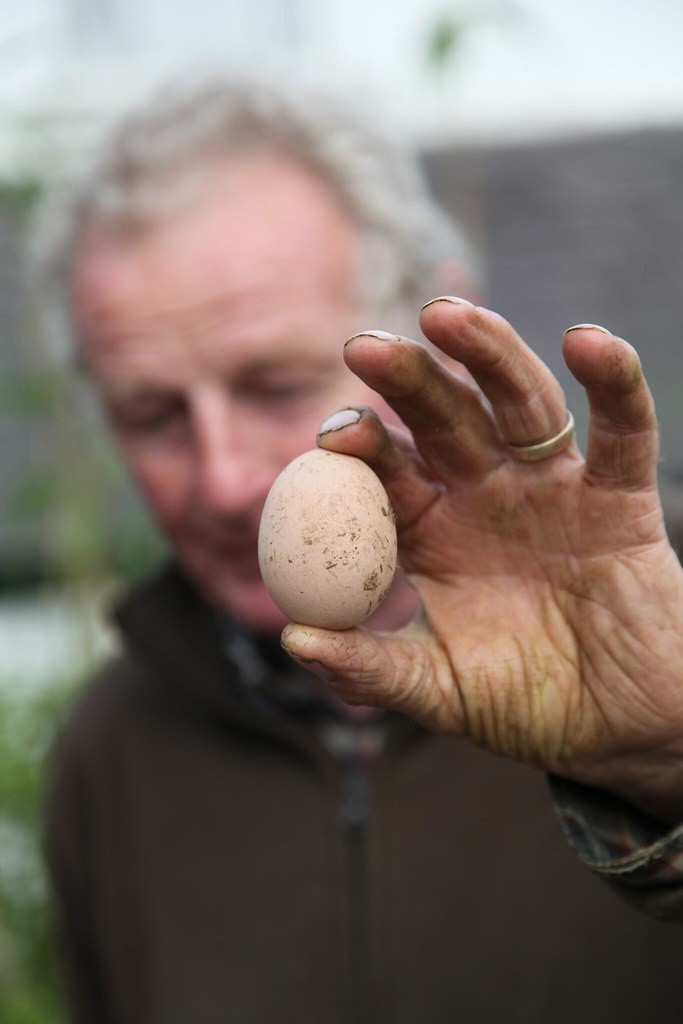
40,76,682,1024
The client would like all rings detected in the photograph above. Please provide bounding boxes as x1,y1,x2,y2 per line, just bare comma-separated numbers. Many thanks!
506,410,576,460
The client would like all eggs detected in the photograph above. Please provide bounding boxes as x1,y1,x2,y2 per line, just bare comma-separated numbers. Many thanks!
258,449,398,631
